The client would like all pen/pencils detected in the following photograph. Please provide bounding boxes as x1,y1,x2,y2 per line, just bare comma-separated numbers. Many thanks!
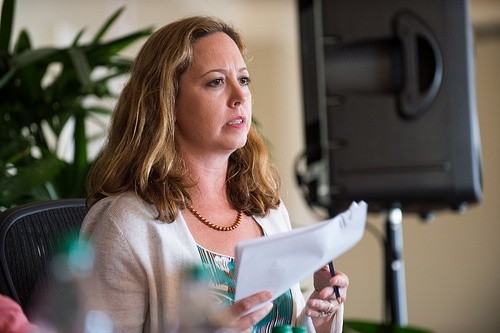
328,261,342,304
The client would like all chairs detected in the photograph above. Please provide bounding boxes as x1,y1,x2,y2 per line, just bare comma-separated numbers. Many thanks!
0,198,107,329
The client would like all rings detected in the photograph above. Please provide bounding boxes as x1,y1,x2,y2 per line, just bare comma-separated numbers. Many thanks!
325,300,334,316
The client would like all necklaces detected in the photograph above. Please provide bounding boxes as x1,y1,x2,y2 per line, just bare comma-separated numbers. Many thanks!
182,196,242,230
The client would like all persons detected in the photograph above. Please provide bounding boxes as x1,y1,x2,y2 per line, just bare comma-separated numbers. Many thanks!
27,16,350,333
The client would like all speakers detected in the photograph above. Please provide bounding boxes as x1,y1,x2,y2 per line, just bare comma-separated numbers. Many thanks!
297,0,483,220
